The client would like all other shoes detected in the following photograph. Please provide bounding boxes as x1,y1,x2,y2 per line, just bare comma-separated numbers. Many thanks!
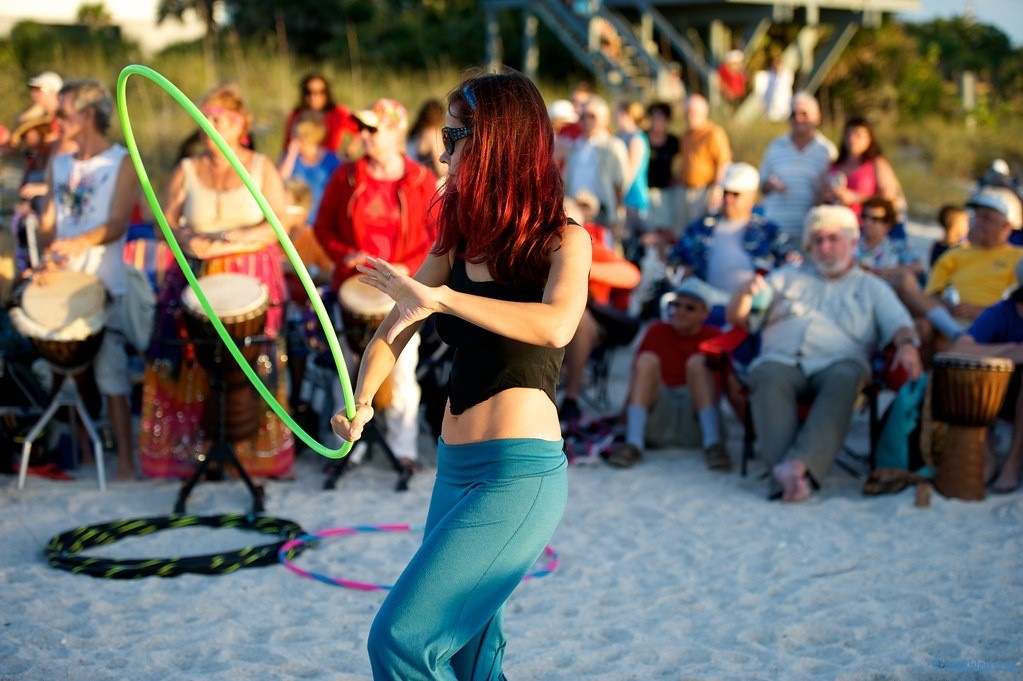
323,458,356,474
861,469,910,495
558,400,581,421
608,444,643,467
399,457,422,474
707,444,731,469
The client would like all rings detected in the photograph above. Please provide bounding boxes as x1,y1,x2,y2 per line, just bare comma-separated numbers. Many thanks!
385,272,391,279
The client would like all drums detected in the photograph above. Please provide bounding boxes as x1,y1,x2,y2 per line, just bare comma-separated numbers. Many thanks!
933,353,1014,500
340,276,395,410
181,272,270,443
10,267,116,424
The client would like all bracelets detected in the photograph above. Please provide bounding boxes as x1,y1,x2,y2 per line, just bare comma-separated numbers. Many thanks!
898,337,922,348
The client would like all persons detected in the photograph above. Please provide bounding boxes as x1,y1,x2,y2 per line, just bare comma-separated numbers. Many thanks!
0,71,208,487
269,70,452,492
724,204,924,502
328,76,593,681
550,39,1023,503
154,83,293,480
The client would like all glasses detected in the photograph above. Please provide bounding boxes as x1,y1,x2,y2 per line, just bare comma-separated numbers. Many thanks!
442,126,473,155
864,215,884,222
55,109,79,119
671,301,706,310
358,121,377,135
723,191,739,197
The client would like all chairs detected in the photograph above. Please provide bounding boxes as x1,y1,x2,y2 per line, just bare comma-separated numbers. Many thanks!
732,322,903,487
556,354,618,418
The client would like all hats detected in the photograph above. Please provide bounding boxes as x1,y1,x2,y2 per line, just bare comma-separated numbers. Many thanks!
722,162,759,193
349,99,410,133
678,278,708,306
550,100,580,124
965,185,1022,230
28,71,63,92
